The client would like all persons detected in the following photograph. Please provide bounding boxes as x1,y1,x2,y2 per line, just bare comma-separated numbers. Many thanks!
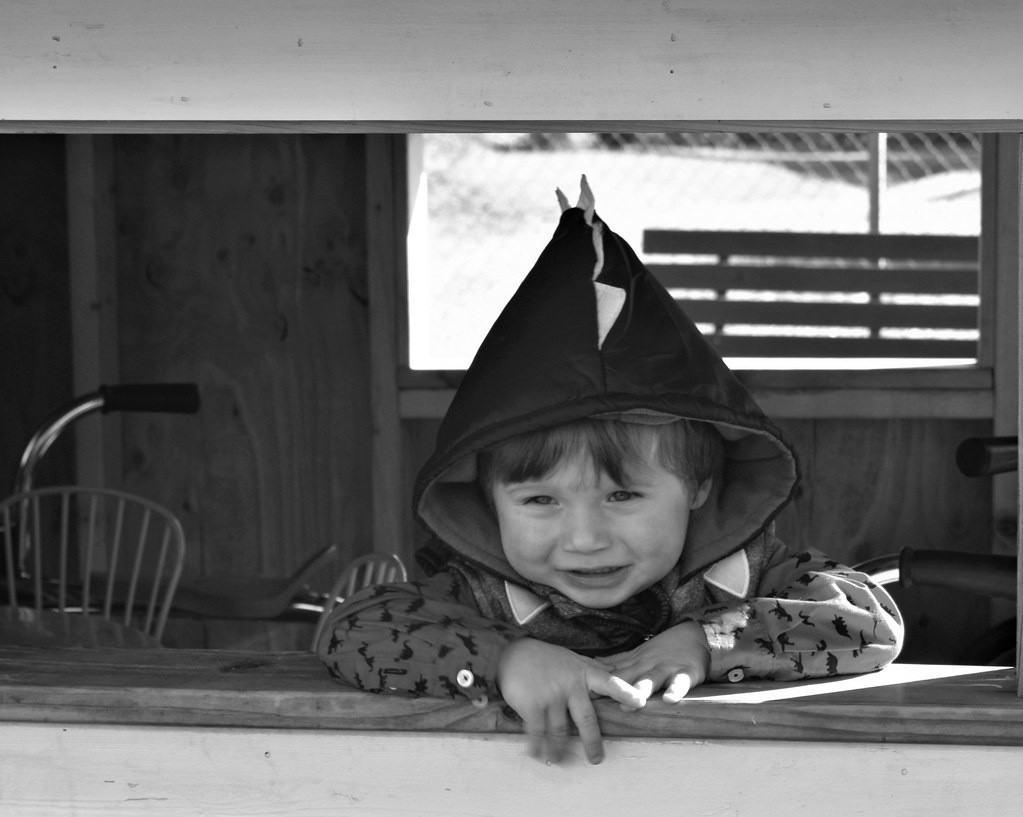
312,175,905,764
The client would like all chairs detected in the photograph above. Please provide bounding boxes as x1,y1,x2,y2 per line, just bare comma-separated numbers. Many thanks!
311,551,407,653
1,484,187,651
